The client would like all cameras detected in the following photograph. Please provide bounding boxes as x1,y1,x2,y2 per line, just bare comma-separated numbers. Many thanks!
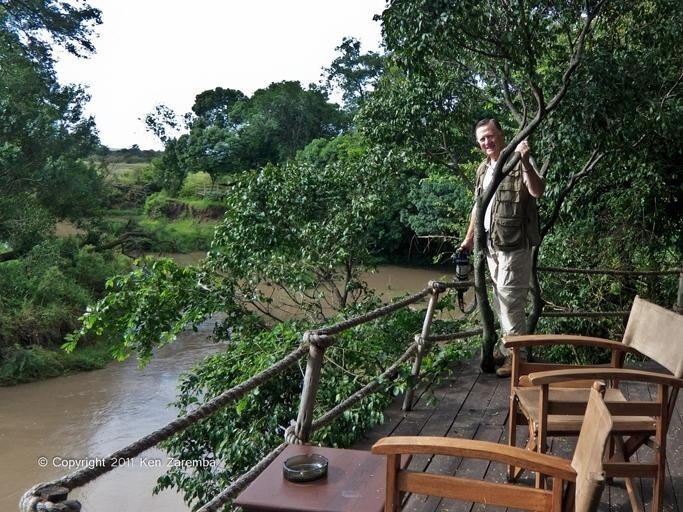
453,250,472,293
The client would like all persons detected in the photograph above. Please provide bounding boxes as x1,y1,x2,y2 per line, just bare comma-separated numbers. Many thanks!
453,119,546,377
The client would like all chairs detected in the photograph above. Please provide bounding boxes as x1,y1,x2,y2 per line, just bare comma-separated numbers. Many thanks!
502,295,682,511
371,379,612,511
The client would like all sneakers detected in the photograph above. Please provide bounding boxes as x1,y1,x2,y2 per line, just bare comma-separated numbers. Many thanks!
493,351,529,376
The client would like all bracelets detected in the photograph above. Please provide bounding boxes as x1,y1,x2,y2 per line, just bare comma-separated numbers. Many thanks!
522,163,532,172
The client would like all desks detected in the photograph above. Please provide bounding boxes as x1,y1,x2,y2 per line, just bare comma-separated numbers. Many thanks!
234,444,387,511
